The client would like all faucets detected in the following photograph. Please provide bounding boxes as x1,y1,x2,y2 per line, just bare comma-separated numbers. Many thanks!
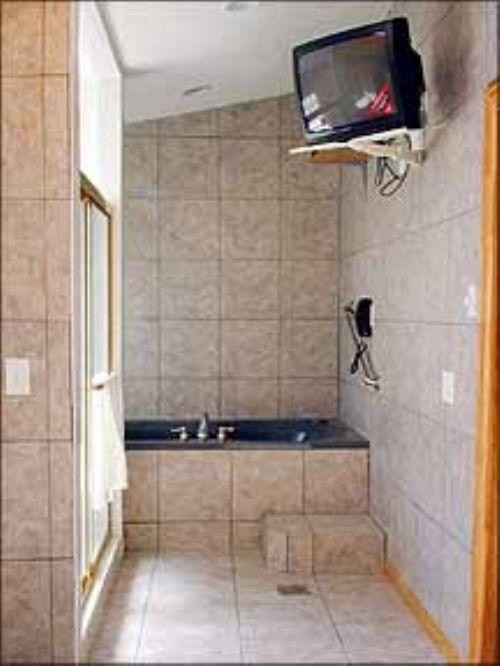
197,413,210,442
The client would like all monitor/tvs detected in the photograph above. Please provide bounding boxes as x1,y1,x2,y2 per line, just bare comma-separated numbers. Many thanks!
292,16,426,146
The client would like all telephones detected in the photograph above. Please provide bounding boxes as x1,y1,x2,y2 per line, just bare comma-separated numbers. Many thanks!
356,298,374,337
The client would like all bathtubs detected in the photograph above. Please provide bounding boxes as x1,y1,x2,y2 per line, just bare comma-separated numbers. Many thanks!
124,419,371,557
